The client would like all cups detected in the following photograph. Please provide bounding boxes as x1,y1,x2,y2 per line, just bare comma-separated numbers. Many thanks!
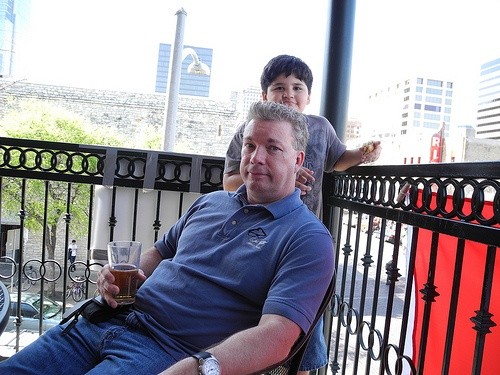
107,241,143,304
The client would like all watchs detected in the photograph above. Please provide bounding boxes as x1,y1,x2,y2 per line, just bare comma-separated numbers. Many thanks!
192,352,223,375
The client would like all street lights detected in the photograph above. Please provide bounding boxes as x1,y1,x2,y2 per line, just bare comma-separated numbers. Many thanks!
162,7,209,155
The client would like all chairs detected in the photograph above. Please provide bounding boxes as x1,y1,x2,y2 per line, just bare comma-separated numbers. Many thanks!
253,266,336,375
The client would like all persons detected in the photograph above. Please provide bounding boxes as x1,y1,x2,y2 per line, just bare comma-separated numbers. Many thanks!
223,55,382,375
68,240,78,272
0,102,336,375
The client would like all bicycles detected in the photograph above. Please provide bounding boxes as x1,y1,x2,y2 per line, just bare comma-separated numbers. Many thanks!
61,277,85,303
12,264,38,291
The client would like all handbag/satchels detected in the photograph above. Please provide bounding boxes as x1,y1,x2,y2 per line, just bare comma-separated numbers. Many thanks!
58,294,129,336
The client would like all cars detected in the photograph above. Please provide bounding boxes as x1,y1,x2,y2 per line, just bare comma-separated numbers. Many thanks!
1,291,72,333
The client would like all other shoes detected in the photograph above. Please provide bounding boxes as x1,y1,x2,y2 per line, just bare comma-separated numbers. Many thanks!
71,267,76,272
